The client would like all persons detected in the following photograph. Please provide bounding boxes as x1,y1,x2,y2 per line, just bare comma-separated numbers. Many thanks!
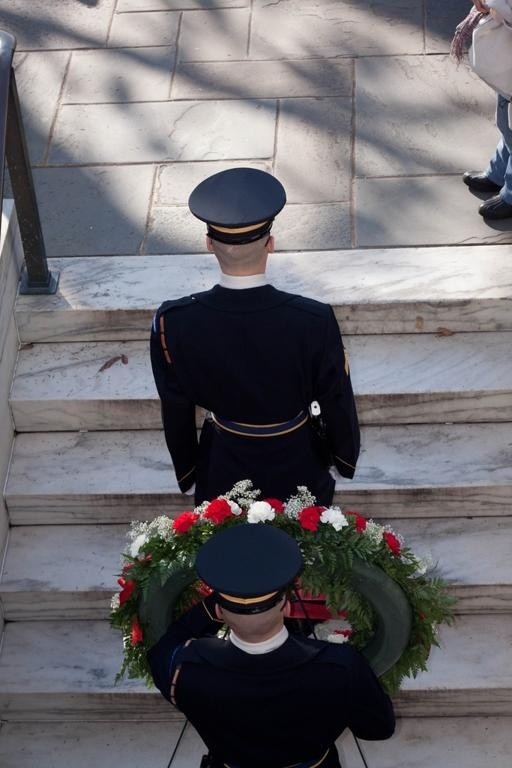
149,168,360,509
449,0,512,220
146,524,396,768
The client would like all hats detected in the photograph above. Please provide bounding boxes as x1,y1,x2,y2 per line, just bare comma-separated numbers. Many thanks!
193,523,306,614
187,166,288,245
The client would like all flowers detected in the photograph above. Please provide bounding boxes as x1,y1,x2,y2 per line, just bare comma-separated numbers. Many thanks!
108,479,459,699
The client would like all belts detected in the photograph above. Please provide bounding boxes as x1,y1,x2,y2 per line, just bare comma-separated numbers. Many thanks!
210,408,309,438
223,747,334,767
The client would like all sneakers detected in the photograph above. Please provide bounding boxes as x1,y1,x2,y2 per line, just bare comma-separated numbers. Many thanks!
462,170,503,193
479,195,512,220
